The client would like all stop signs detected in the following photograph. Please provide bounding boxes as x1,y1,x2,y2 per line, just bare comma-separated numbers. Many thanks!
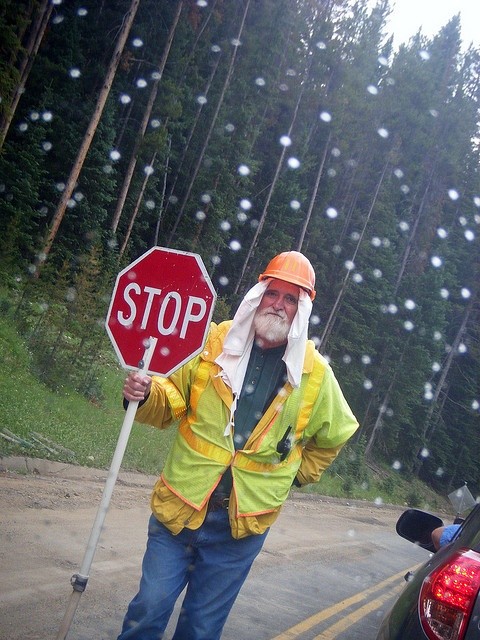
104,246,217,379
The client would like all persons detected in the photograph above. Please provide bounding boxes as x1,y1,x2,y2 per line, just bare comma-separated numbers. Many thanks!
114,250,361,639
431,525,462,553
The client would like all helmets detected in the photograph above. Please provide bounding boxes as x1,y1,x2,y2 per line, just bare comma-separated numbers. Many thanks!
258,251,316,300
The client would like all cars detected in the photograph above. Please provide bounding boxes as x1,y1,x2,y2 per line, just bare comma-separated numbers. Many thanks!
375,503,480,640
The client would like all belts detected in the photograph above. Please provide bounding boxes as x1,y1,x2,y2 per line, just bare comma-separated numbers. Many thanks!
209,492,229,510
